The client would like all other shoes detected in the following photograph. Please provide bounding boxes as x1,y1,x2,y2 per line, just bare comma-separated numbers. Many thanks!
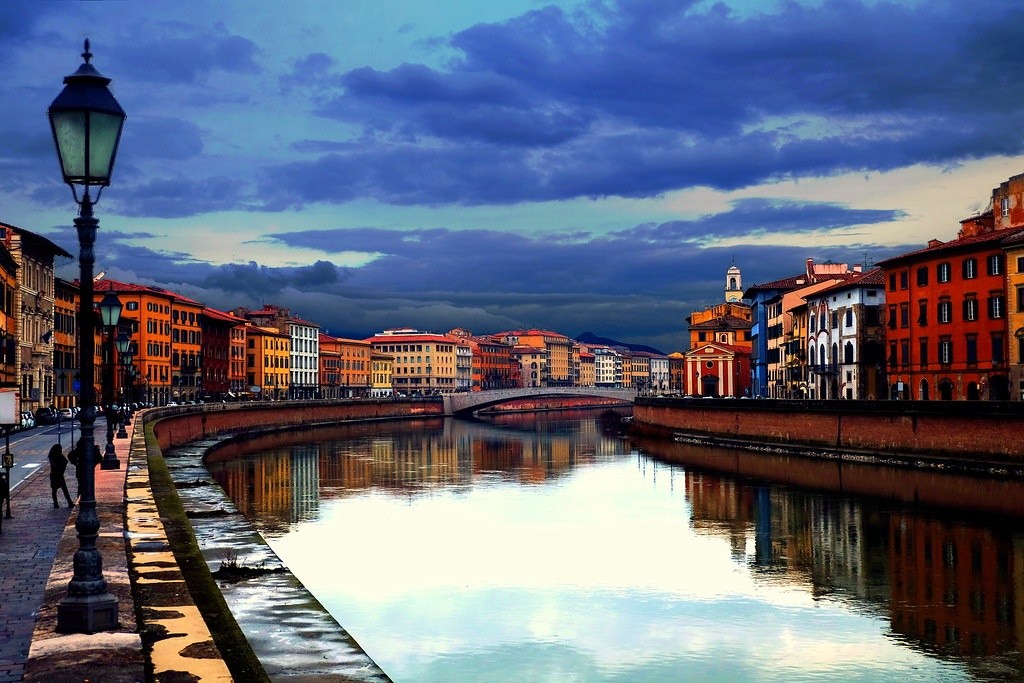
54,504,59,508
69,504,75,508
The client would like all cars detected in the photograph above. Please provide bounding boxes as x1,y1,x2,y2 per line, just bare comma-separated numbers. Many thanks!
20,406,80,430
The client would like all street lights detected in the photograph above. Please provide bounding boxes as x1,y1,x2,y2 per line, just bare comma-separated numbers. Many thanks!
178,379,182,404
116,332,150,439
48,37,127,637
100,280,120,470
161,376,165,406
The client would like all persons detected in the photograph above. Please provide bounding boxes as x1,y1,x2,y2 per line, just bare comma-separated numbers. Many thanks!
112,401,136,430
47,444,75,509
